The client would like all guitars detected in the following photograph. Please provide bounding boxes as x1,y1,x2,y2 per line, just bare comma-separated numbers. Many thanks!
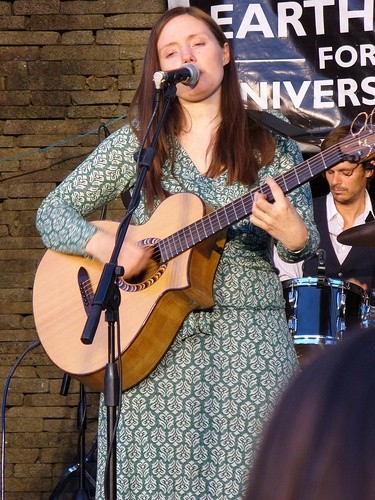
33,123,375,394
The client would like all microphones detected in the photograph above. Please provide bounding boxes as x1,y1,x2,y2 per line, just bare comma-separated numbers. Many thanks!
154,63,200,86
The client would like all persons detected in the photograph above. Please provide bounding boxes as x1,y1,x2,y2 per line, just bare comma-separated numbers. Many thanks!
273,123,374,306
241,325,375,500
33,6,316,500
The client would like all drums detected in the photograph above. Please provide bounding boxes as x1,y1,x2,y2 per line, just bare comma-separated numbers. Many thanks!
280,275,371,348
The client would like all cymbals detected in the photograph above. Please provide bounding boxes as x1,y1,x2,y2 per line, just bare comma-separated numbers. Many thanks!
336,221,375,248
